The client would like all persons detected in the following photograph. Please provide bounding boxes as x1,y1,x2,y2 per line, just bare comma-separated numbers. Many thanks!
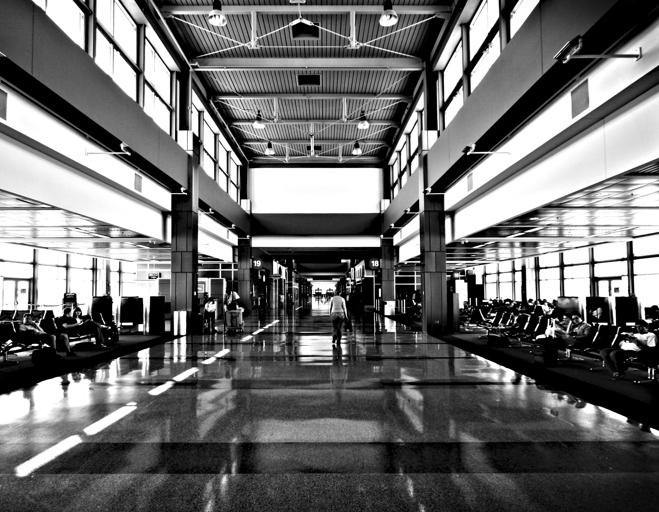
459,294,659,384
278,289,285,316
329,343,348,404
225,334,241,378
253,294,266,325
1,311,56,357
328,290,348,344
203,291,210,304
203,295,218,333
342,289,355,332
203,334,218,375
38,310,75,358
285,323,301,365
59,307,92,338
286,293,294,319
348,334,363,365
302,290,343,301
72,308,107,350
223,286,242,334
30,309,41,323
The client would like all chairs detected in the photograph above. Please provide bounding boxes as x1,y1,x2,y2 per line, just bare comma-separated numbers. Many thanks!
485,310,659,384
0,310,97,362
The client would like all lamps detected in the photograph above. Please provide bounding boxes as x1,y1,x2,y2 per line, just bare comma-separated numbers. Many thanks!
422,187,445,196
207,0,399,27
252,109,370,155
380,208,420,241
553,33,641,65
461,143,510,158
86,142,251,241
